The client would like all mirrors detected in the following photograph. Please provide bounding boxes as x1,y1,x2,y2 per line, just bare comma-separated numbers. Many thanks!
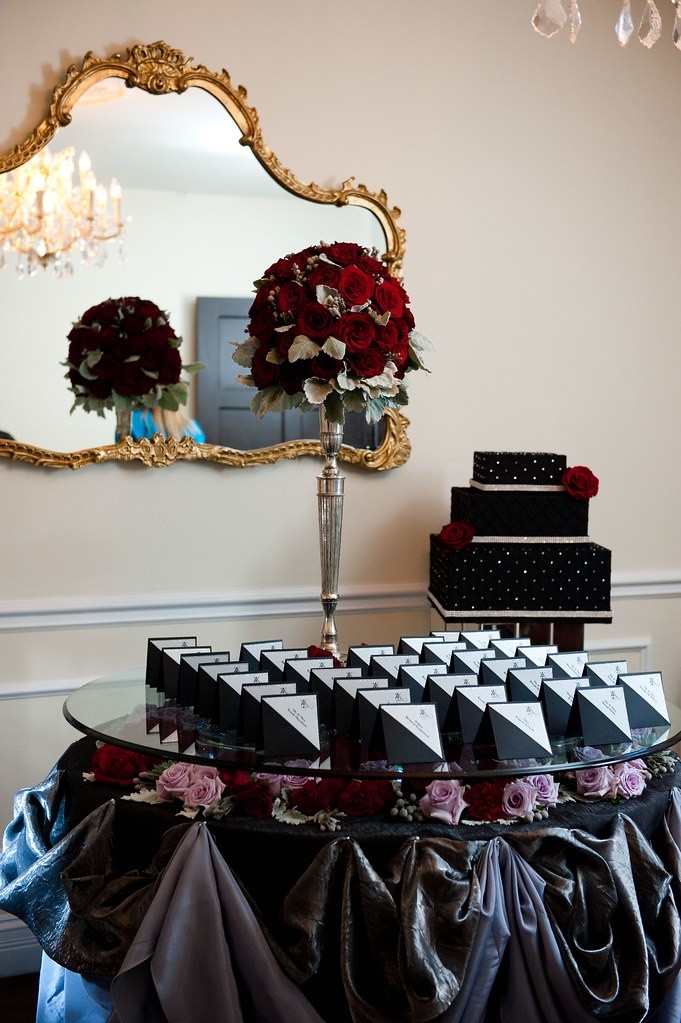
0,41,412,473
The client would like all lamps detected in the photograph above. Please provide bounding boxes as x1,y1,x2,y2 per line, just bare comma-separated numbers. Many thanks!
0,148,128,279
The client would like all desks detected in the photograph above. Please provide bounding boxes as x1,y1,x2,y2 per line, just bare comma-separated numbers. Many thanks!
30,662,681,1023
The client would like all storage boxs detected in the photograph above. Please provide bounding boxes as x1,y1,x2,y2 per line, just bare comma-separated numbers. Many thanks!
425,451,613,624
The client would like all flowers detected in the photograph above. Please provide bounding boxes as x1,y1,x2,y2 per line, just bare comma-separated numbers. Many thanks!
79,728,678,827
438,521,476,551
561,466,599,499
60,296,206,419
233,242,432,425
